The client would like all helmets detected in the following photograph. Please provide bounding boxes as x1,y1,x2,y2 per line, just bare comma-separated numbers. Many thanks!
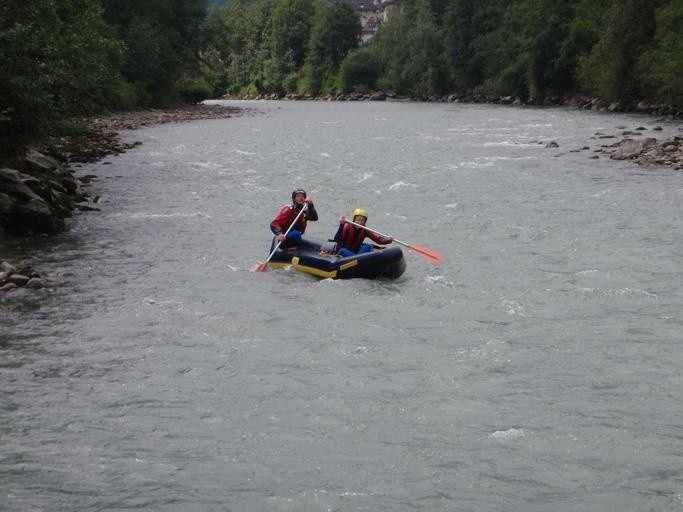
352,208,369,226
292,188,307,204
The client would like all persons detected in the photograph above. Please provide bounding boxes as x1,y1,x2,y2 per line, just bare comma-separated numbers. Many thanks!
270,189,318,249
334,208,393,258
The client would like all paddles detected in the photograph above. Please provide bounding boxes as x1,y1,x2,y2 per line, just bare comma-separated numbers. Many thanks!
343,218,441,264
255,203,307,272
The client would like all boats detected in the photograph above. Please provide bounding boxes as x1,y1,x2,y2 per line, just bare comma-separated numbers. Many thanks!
268,235,408,281
